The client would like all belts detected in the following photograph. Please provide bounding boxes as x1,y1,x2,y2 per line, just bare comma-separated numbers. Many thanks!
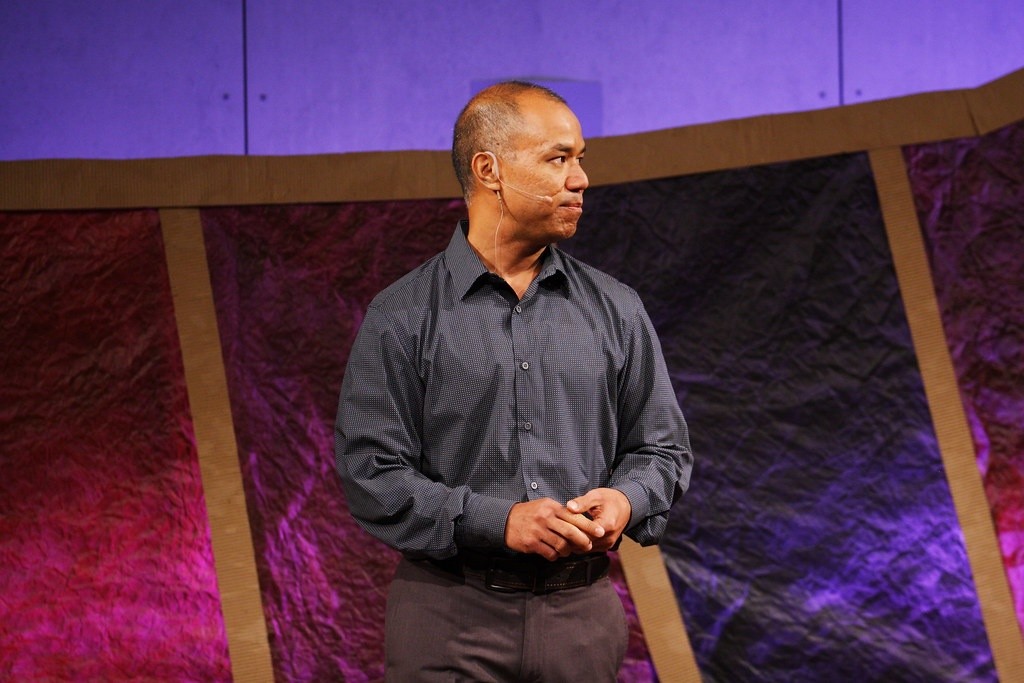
404,544,610,593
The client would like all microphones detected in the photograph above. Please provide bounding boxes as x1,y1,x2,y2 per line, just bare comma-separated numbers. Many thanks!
496,172,553,204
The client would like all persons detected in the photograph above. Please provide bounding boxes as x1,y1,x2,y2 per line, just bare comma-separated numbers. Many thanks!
333,80,693,683
